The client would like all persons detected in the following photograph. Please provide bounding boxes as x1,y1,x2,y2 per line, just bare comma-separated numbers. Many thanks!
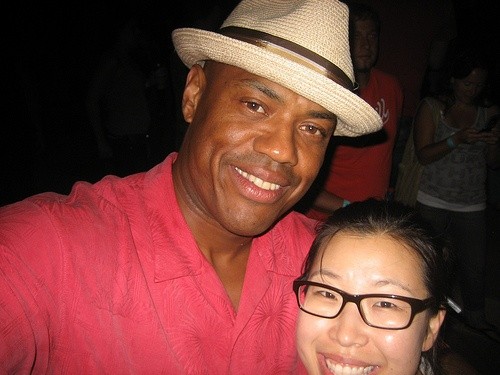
407,49,500,333
306,4,403,218
0,1,383,375
292,195,460,375
85,15,161,168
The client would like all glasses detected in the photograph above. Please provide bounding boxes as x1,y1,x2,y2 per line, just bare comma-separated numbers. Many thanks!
291,274,435,329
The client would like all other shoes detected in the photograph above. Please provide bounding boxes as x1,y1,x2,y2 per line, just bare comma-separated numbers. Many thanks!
464,316,500,342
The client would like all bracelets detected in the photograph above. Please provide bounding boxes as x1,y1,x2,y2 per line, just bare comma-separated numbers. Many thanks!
343,198,350,208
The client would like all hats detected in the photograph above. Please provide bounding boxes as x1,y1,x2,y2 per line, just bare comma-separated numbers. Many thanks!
171,0,384,136
